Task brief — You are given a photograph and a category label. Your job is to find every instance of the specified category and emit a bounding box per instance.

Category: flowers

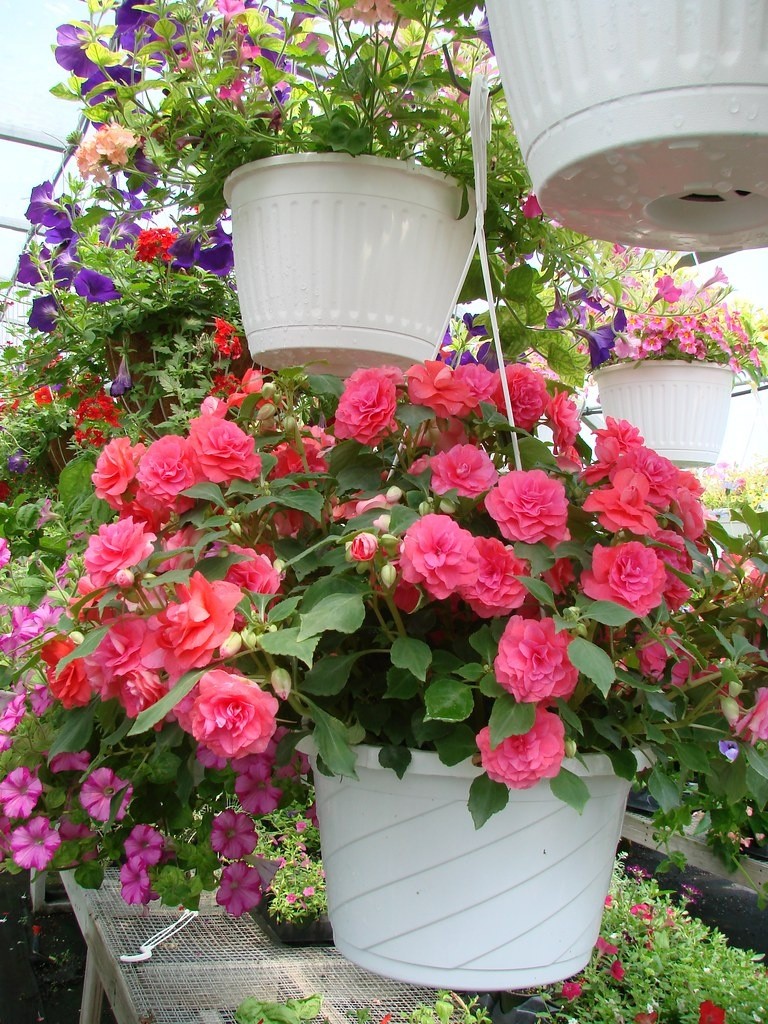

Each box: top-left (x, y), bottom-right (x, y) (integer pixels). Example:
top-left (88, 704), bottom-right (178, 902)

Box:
top-left (1, 0), bottom-right (766, 1023)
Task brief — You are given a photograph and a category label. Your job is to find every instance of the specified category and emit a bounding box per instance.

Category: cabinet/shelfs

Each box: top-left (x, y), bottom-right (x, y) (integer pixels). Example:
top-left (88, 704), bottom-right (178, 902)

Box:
top-left (625, 785), bottom-right (768, 895)
top-left (26, 859), bottom-right (483, 1023)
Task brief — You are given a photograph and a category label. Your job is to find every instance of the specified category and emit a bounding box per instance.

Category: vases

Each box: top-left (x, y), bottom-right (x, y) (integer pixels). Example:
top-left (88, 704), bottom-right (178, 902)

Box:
top-left (483, 0), bottom-right (766, 253)
top-left (222, 152), bottom-right (477, 379)
top-left (292, 732), bottom-right (659, 992)
top-left (102, 322), bottom-right (260, 442)
top-left (257, 885), bottom-right (334, 945)
top-left (46, 424), bottom-right (82, 472)
top-left (593, 358), bottom-right (735, 470)
